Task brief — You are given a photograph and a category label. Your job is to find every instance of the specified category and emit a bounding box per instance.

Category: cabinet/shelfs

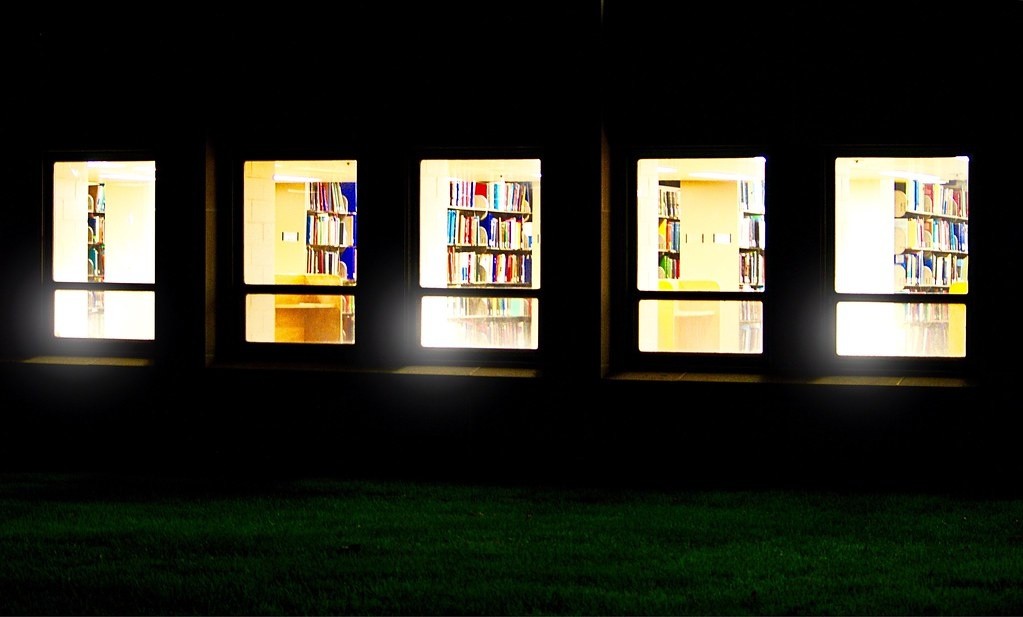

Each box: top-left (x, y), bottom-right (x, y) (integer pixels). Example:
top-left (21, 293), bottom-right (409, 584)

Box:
top-left (659, 179), bottom-right (682, 280)
top-left (892, 172), bottom-right (969, 359)
top-left (737, 177), bottom-right (764, 354)
top-left (87, 180), bottom-right (108, 319)
top-left (273, 273), bottom-right (342, 344)
top-left (448, 176), bottom-right (538, 350)
top-left (305, 180), bottom-right (357, 343)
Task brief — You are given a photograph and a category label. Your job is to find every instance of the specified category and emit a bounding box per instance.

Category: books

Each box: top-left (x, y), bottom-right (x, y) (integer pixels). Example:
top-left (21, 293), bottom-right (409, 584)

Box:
top-left (88, 184), bottom-right (104, 314)
top-left (448, 178), bottom-right (535, 343)
top-left (307, 182), bottom-right (356, 279)
top-left (891, 182), bottom-right (969, 355)
top-left (740, 182), bottom-right (765, 353)
top-left (658, 182), bottom-right (682, 286)
